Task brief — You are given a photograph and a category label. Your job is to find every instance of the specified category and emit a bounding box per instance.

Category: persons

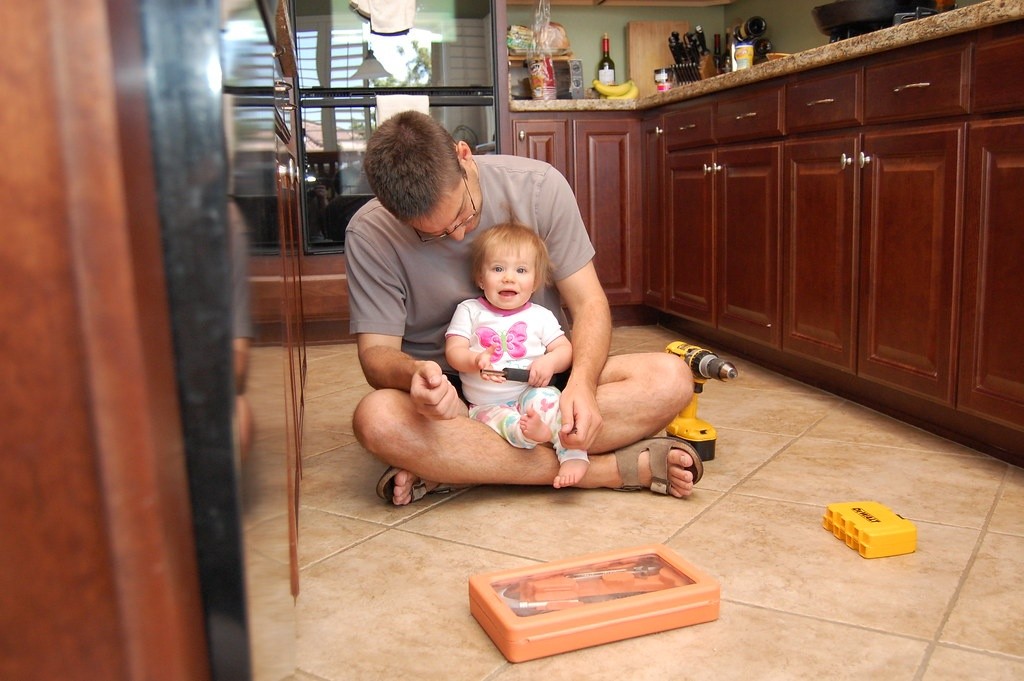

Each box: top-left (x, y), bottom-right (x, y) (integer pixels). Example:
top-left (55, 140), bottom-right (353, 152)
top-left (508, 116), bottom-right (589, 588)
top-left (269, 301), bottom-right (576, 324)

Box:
top-left (230, 197), bottom-right (255, 460)
top-left (343, 112), bottom-right (703, 504)
top-left (305, 181), bottom-right (332, 241)
top-left (445, 224), bottom-right (588, 488)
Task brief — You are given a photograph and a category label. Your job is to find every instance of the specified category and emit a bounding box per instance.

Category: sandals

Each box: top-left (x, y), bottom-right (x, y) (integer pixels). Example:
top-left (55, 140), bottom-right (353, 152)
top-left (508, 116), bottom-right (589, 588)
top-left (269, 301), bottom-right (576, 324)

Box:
top-left (377, 468), bottom-right (468, 503)
top-left (613, 437), bottom-right (704, 494)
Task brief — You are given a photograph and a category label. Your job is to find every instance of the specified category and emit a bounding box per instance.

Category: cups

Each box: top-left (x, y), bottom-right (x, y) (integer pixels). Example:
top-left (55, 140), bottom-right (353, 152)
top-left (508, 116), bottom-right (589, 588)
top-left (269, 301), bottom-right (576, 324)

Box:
top-left (655, 69), bottom-right (674, 92)
top-left (735, 42), bottom-right (753, 70)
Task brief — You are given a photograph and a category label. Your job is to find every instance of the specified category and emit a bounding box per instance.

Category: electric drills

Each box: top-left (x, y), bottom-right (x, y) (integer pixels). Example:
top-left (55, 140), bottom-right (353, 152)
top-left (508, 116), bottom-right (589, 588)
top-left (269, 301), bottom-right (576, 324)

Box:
top-left (661, 341), bottom-right (737, 462)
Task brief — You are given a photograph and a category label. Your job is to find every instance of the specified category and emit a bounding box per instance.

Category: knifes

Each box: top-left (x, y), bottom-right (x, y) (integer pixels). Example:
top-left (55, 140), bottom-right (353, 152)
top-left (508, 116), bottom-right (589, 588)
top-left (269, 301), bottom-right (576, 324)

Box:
top-left (668, 26), bottom-right (710, 85)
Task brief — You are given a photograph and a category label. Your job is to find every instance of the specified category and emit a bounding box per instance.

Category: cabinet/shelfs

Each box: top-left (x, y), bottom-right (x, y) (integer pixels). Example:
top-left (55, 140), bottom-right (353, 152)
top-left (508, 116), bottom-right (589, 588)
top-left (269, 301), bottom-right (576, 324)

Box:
top-left (507, 108), bottom-right (641, 322)
top-left (640, 16), bottom-right (1023, 471)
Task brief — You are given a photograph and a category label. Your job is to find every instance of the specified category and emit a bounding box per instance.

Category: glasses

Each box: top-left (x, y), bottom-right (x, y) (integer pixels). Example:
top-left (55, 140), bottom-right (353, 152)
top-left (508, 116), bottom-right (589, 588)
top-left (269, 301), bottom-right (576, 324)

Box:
top-left (412, 157), bottom-right (479, 242)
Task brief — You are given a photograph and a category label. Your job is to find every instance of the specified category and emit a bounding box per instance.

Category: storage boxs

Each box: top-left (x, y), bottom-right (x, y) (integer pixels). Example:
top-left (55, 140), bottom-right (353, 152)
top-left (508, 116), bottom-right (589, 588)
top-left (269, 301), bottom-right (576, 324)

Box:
top-left (467, 542), bottom-right (721, 664)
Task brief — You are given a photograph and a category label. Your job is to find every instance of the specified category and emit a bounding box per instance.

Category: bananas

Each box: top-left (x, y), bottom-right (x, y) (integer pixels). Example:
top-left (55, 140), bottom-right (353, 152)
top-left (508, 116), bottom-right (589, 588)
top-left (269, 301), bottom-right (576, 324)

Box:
top-left (593, 79), bottom-right (639, 100)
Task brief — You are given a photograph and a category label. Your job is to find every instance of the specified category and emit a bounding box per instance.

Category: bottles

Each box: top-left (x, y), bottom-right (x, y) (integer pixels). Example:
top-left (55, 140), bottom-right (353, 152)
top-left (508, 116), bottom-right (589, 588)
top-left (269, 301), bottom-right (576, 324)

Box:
top-left (599, 33), bottom-right (614, 85)
top-left (754, 39), bottom-right (772, 60)
top-left (722, 28), bottom-right (732, 73)
top-left (713, 34), bottom-right (721, 74)
top-left (734, 16), bottom-right (767, 42)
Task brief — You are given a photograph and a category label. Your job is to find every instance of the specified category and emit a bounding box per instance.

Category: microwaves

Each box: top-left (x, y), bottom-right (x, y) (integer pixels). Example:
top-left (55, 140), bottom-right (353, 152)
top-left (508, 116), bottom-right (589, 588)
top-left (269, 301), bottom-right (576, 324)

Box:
top-left (510, 57), bottom-right (583, 99)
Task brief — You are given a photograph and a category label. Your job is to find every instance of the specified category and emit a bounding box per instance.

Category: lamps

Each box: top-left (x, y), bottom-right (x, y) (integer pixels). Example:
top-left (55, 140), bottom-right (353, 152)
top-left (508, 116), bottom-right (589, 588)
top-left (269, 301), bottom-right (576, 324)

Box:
top-left (348, 33), bottom-right (390, 80)
